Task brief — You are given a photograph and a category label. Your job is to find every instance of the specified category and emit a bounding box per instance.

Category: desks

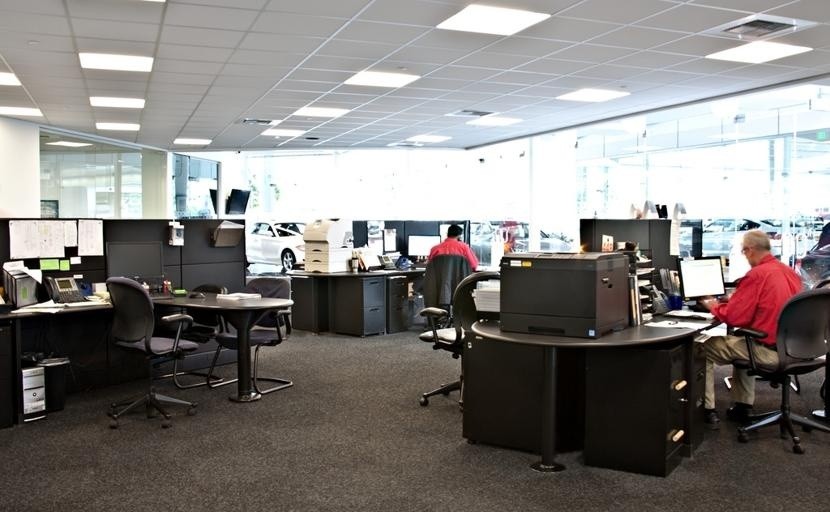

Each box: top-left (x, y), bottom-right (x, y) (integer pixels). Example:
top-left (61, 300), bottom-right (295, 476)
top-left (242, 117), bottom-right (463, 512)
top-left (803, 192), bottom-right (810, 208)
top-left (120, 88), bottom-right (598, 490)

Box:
top-left (0, 291), bottom-right (294, 429)
top-left (462, 300), bottom-right (723, 473)
top-left (287, 266), bottom-right (425, 339)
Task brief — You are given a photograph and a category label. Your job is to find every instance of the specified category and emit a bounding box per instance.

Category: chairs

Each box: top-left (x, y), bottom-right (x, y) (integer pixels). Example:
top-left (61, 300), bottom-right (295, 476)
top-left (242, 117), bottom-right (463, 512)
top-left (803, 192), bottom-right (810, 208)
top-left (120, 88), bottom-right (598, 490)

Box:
top-left (732, 285), bottom-right (830, 455)
top-left (155, 283), bottom-right (228, 389)
top-left (423, 256), bottom-right (472, 330)
top-left (106, 276), bottom-right (197, 428)
top-left (206, 276), bottom-right (294, 394)
top-left (418, 271), bottom-right (501, 408)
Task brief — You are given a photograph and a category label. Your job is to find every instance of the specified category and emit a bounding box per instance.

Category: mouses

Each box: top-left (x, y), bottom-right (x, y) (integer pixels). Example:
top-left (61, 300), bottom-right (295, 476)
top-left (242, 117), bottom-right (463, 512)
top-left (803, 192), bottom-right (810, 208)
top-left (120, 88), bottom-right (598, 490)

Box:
top-left (189, 292), bottom-right (205, 299)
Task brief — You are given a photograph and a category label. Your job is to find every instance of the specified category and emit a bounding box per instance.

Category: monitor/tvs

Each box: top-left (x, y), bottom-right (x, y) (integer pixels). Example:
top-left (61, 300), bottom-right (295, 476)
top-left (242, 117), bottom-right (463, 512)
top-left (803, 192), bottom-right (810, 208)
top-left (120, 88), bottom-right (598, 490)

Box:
top-left (676, 255), bottom-right (725, 311)
top-left (106, 240), bottom-right (164, 289)
top-left (226, 188), bottom-right (251, 214)
top-left (408, 234), bottom-right (441, 261)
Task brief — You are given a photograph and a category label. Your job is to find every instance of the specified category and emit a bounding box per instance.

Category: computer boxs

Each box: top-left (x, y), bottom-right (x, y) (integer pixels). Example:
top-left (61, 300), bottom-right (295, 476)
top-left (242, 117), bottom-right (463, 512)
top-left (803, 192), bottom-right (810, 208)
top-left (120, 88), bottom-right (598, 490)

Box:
top-left (21, 366), bottom-right (47, 423)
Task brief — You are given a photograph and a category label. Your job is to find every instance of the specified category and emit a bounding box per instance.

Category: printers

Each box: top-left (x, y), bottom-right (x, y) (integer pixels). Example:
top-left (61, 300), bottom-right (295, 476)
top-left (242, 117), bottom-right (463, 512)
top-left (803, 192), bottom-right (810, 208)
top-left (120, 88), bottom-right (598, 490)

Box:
top-left (498, 252), bottom-right (631, 340)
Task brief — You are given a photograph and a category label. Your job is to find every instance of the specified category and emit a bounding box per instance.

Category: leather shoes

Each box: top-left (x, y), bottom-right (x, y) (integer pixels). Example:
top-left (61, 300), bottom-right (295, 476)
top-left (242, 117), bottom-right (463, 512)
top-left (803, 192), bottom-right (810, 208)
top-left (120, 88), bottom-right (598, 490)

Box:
top-left (727, 405), bottom-right (751, 418)
top-left (705, 413), bottom-right (721, 431)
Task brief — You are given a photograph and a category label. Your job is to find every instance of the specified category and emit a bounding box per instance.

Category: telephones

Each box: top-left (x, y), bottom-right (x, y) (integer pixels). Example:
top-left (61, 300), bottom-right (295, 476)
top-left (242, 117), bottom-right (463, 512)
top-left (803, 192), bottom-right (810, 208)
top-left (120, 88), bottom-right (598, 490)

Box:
top-left (640, 284), bottom-right (669, 315)
top-left (378, 255), bottom-right (397, 270)
top-left (43, 275), bottom-right (85, 303)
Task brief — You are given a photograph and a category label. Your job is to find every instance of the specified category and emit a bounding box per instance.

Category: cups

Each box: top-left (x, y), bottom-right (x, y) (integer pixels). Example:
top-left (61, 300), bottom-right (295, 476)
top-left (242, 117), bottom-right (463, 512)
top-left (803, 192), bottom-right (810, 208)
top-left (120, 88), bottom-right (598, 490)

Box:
top-left (667, 294), bottom-right (682, 310)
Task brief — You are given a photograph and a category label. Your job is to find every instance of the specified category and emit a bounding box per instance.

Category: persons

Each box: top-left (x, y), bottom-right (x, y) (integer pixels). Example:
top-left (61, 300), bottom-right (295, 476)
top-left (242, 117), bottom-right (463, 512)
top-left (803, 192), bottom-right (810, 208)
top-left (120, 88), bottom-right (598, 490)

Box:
top-left (703, 229), bottom-right (804, 431)
top-left (429, 225), bottom-right (480, 269)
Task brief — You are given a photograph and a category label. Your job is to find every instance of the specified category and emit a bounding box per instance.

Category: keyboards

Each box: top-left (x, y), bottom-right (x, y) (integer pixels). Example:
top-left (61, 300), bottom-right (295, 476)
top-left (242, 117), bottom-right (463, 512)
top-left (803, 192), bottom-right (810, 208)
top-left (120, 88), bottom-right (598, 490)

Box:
top-left (149, 292), bottom-right (173, 300)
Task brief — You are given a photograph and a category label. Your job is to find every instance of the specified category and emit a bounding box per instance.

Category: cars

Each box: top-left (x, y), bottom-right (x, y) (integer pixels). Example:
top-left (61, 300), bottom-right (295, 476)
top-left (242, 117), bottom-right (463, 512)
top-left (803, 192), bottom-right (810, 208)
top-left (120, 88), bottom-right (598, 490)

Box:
top-left (469, 222), bottom-right (582, 265)
top-left (677, 215), bottom-right (830, 289)
top-left (246, 220), bottom-right (315, 271)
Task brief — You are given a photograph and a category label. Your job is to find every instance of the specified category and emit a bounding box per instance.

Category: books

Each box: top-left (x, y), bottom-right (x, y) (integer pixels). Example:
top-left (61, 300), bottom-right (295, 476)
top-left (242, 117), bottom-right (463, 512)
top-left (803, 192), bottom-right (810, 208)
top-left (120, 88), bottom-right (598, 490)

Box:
top-left (629, 267), bottom-right (655, 326)
top-left (471, 289), bottom-right (501, 312)
top-left (217, 291), bottom-right (260, 301)
